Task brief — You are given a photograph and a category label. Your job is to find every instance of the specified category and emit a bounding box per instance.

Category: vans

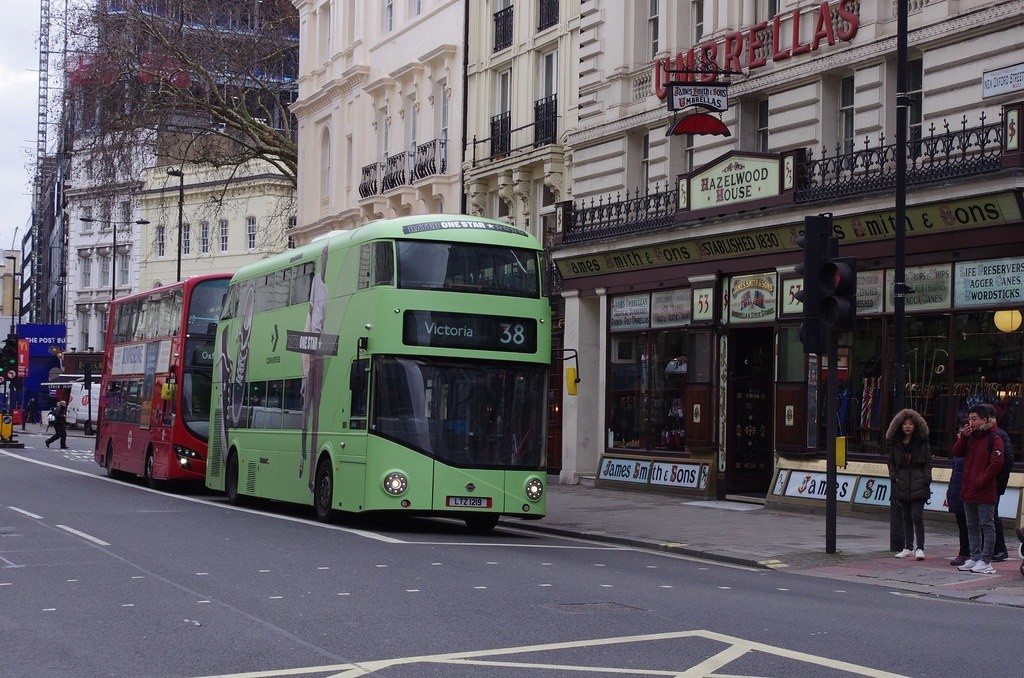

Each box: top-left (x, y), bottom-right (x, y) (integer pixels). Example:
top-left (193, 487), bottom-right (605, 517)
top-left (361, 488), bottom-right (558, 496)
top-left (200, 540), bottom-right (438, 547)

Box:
top-left (65, 381), bottom-right (101, 431)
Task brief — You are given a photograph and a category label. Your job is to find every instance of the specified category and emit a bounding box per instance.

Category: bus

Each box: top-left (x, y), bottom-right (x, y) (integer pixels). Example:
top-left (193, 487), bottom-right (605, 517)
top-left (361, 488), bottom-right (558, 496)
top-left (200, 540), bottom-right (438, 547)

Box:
top-left (94, 272), bottom-right (234, 491)
top-left (206, 214), bottom-right (581, 534)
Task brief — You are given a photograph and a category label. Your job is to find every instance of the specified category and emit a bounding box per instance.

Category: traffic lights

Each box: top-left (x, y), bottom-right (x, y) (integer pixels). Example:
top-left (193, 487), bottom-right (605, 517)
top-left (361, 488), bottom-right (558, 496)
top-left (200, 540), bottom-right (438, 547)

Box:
top-left (6, 339), bottom-right (18, 369)
top-left (819, 256), bottom-right (857, 332)
top-left (0, 359), bottom-right (6, 375)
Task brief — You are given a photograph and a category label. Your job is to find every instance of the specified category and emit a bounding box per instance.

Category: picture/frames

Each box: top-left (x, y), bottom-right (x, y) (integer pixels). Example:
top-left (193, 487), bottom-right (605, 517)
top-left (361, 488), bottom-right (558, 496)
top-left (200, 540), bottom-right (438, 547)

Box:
top-left (615, 337), bottom-right (635, 363)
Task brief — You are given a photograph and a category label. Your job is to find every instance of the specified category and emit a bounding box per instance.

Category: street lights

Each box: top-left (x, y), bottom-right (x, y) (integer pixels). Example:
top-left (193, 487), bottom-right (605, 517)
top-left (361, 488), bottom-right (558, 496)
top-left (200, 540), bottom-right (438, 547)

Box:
top-left (6, 254), bottom-right (17, 333)
top-left (80, 217), bottom-right (151, 302)
top-left (166, 170), bottom-right (184, 283)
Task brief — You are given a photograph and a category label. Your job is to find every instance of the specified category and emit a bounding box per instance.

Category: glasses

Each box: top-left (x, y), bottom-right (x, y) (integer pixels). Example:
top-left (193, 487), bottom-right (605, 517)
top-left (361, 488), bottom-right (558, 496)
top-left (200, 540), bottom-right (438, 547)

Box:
top-left (968, 418), bottom-right (982, 421)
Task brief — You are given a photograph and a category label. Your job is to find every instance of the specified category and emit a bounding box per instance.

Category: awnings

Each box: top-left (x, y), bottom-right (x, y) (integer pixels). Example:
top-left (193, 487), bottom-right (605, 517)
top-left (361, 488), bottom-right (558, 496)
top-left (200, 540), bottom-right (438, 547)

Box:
top-left (42, 373), bottom-right (102, 386)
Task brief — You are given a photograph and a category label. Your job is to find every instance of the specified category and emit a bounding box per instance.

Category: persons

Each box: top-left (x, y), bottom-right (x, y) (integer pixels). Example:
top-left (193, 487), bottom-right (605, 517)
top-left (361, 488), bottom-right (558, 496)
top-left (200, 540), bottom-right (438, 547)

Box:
top-left (192, 394), bottom-right (203, 410)
top-left (2, 398), bottom-right (36, 424)
top-left (946, 404), bottom-right (1013, 574)
top-left (885, 408), bottom-right (933, 561)
top-left (55, 385), bottom-right (64, 401)
top-left (45, 401), bottom-right (69, 449)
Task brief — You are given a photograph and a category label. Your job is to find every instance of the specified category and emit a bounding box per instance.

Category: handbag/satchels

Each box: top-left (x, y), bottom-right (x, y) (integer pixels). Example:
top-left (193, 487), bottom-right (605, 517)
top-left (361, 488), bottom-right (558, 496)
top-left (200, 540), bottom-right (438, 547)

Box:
top-left (47, 413), bottom-right (57, 426)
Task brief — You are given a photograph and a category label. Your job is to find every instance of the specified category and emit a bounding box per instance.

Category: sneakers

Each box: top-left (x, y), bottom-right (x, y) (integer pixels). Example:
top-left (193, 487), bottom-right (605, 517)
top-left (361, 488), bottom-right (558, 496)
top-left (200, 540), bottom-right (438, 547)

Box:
top-left (950, 556), bottom-right (969, 565)
top-left (970, 560), bottom-right (992, 572)
top-left (916, 549), bottom-right (925, 559)
top-left (990, 551), bottom-right (1008, 561)
top-left (61, 446), bottom-right (69, 449)
top-left (895, 549), bottom-right (915, 558)
top-left (958, 559), bottom-right (976, 570)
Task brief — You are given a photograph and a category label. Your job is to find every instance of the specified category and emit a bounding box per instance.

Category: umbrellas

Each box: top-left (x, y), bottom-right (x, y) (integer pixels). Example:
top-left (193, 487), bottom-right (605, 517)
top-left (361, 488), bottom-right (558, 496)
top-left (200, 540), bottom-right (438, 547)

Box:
top-left (838, 375), bottom-right (1023, 450)
top-left (614, 395), bottom-right (684, 447)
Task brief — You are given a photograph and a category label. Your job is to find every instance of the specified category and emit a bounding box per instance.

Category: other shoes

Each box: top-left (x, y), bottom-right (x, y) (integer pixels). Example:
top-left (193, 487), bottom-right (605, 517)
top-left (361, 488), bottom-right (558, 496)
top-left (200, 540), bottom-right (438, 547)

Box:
top-left (45, 440), bottom-right (49, 447)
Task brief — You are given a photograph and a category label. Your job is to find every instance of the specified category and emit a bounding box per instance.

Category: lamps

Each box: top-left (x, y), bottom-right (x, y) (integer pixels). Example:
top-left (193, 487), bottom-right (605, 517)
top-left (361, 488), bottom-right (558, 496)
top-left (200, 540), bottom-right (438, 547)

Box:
top-left (994, 309), bottom-right (1022, 333)
